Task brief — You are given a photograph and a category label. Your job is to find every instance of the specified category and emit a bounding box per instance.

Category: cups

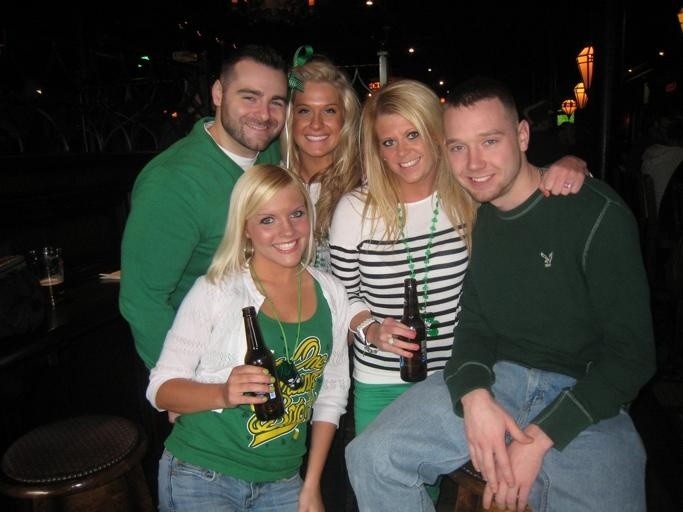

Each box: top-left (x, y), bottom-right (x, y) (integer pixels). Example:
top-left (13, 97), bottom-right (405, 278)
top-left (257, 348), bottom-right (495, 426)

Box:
top-left (27, 247), bottom-right (65, 306)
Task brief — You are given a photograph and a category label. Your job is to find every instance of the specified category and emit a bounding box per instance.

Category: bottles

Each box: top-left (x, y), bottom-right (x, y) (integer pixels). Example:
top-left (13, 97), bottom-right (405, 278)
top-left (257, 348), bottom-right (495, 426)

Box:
top-left (242, 306), bottom-right (284, 422)
top-left (399, 278), bottom-right (427, 383)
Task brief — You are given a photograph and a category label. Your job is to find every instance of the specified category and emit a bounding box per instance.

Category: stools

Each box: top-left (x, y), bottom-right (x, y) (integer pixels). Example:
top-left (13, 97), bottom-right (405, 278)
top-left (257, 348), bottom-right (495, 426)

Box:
top-left (0, 412), bottom-right (158, 512)
top-left (447, 463), bottom-right (536, 512)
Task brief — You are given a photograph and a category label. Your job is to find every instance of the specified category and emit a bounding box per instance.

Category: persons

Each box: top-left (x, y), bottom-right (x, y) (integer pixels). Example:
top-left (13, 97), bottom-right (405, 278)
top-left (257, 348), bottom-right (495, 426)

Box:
top-left (115, 47), bottom-right (683, 512)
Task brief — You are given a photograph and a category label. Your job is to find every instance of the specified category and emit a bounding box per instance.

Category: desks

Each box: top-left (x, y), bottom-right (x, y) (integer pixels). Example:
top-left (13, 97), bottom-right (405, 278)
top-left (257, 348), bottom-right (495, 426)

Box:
top-left (0, 257), bottom-right (120, 368)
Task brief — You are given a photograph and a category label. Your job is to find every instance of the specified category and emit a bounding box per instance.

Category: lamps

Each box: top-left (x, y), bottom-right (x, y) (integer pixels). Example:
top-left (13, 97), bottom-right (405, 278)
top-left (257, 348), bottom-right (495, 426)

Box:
top-left (575, 40), bottom-right (601, 92)
top-left (561, 99), bottom-right (577, 121)
top-left (573, 82), bottom-right (589, 111)
